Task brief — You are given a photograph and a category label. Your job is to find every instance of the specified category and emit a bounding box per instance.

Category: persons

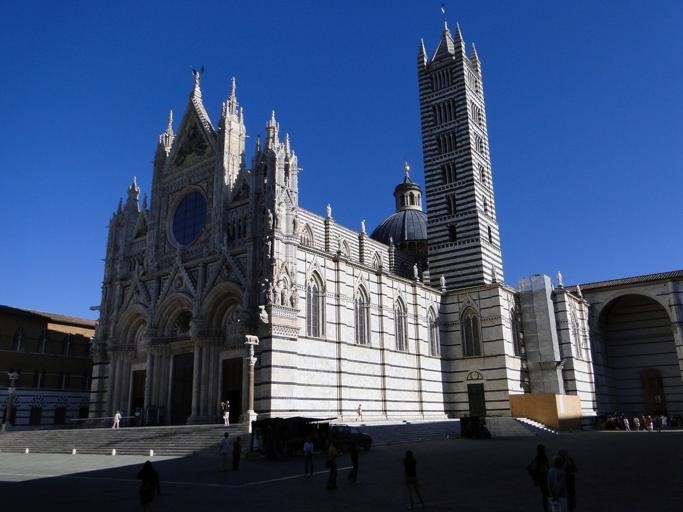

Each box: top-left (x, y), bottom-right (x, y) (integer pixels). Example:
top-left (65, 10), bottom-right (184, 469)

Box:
top-left (403, 450), bottom-right (424, 509)
top-left (113, 410), bottom-right (122, 430)
top-left (218, 432), bottom-right (229, 472)
top-left (300, 434), bottom-right (361, 489)
top-left (233, 437), bottom-right (242, 470)
top-left (530, 443), bottom-right (577, 511)
top-left (136, 461), bottom-right (160, 512)
top-left (221, 401), bottom-right (230, 426)
top-left (605, 411), bottom-right (669, 432)
top-left (355, 404), bottom-right (363, 421)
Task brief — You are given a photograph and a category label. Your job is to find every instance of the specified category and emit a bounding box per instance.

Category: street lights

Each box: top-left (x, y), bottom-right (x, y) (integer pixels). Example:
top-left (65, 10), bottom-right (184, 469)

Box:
top-left (243, 333), bottom-right (262, 435)
top-left (1, 370), bottom-right (21, 434)
